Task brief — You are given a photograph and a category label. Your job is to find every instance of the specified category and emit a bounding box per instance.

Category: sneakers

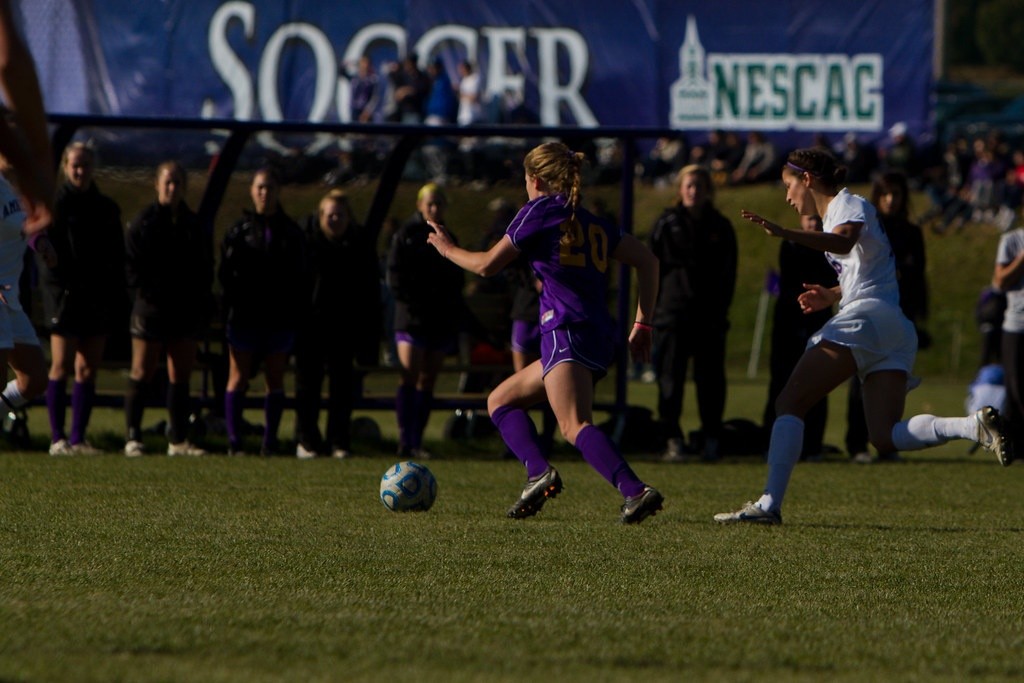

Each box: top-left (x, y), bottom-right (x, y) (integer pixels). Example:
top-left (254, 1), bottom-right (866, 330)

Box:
top-left (506, 465), bottom-right (563, 518)
top-left (621, 485), bottom-right (662, 523)
top-left (978, 406), bottom-right (1013, 466)
top-left (713, 501), bottom-right (782, 524)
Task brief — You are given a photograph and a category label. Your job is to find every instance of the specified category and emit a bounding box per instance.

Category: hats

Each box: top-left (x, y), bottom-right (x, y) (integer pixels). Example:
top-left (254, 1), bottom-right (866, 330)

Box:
top-left (889, 122), bottom-right (908, 136)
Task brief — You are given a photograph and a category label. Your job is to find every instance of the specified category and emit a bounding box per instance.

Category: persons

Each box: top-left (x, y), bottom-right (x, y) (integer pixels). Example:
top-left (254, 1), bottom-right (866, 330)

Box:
top-left (424, 139), bottom-right (666, 524)
top-left (714, 145), bottom-right (1014, 526)
top-left (1, 51), bottom-right (1024, 463)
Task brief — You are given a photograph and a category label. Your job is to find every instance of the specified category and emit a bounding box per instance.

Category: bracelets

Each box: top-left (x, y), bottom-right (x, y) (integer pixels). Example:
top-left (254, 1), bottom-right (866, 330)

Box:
top-left (634, 319), bottom-right (653, 327)
top-left (633, 321), bottom-right (653, 331)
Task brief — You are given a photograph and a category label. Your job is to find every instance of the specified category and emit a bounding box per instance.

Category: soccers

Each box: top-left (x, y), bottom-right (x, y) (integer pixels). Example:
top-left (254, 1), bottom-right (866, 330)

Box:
top-left (379, 461), bottom-right (437, 514)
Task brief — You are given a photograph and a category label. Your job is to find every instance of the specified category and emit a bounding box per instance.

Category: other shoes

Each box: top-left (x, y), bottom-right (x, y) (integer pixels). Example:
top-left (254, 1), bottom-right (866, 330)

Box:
top-left (848, 447), bottom-right (872, 463)
top-left (49, 439), bottom-right (104, 456)
top-left (297, 443), bottom-right (349, 459)
top-left (397, 447), bottom-right (432, 459)
top-left (168, 438), bottom-right (205, 457)
top-left (227, 440), bottom-right (280, 460)
top-left (125, 441), bottom-right (145, 458)
top-left (664, 438), bottom-right (723, 461)
